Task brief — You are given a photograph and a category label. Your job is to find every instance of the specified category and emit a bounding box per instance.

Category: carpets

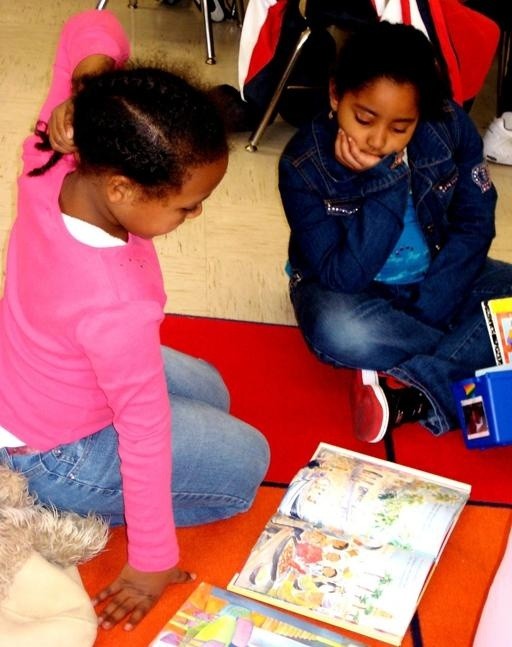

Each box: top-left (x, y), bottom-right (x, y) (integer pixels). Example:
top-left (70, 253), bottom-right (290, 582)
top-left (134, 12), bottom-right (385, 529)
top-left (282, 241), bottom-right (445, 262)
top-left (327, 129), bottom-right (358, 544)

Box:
top-left (80, 307), bottom-right (511, 646)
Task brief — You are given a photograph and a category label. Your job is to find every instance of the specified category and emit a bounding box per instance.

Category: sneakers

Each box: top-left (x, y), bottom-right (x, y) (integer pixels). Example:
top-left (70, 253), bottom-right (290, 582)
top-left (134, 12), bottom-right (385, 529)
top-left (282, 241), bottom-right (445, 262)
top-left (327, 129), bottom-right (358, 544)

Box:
top-left (352, 366), bottom-right (423, 443)
top-left (481, 116), bottom-right (512, 165)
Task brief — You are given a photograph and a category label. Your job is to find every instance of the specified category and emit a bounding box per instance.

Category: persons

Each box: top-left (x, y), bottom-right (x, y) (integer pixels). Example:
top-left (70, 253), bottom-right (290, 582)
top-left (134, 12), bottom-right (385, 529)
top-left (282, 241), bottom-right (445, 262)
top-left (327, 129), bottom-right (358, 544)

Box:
top-left (280, 24), bottom-right (512, 446)
top-left (373, 1), bottom-right (512, 169)
top-left (0, 8), bottom-right (270, 633)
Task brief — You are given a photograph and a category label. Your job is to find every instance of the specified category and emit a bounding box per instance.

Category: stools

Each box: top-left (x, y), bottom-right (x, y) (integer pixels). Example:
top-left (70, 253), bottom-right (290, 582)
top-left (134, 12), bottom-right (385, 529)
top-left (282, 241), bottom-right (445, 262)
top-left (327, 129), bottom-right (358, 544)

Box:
top-left (95, 0), bottom-right (217, 66)
top-left (243, 24), bottom-right (328, 154)
